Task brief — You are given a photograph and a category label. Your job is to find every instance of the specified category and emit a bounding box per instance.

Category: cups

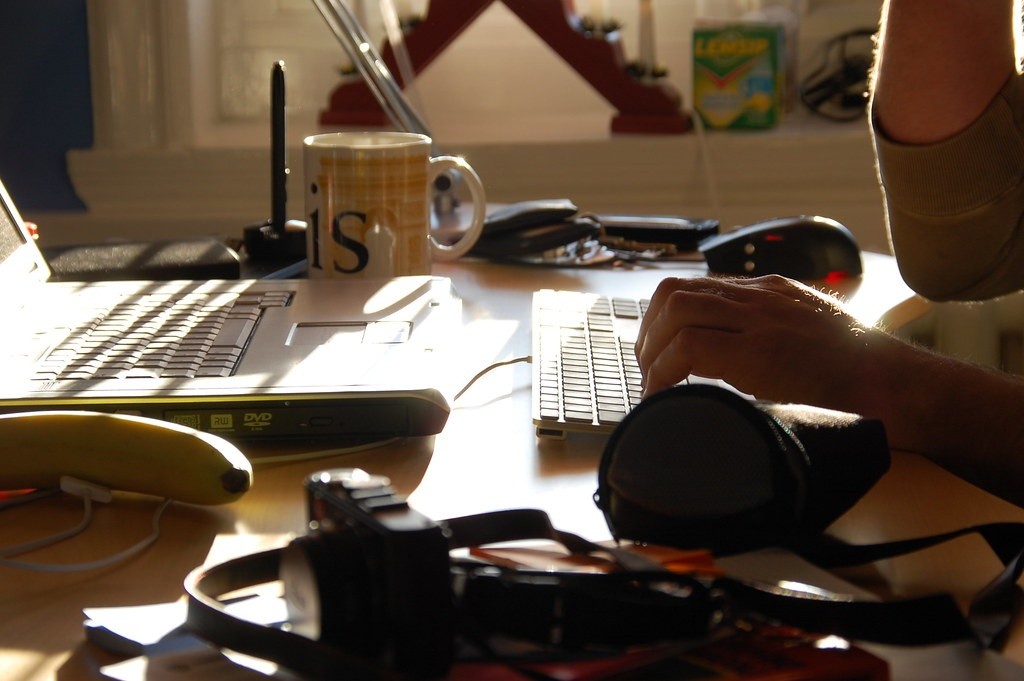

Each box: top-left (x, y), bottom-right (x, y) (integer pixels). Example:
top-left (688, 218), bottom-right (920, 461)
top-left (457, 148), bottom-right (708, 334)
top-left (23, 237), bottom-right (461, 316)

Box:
top-left (303, 132), bottom-right (487, 282)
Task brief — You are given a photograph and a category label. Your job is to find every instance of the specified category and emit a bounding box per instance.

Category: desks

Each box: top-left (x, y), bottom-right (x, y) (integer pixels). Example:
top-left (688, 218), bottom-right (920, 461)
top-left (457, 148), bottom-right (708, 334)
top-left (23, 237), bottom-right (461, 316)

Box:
top-left (0, 249), bottom-right (1024, 681)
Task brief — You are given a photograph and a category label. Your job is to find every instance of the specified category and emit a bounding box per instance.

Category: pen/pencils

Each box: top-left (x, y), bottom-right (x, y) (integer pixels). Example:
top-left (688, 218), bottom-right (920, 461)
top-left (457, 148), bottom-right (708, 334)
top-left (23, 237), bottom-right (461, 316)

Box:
top-left (270, 60), bottom-right (289, 230)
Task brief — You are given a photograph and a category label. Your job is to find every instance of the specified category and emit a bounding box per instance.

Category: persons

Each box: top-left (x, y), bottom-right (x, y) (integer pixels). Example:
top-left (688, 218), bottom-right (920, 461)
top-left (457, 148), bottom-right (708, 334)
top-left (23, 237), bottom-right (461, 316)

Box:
top-left (633, 0), bottom-right (1024, 509)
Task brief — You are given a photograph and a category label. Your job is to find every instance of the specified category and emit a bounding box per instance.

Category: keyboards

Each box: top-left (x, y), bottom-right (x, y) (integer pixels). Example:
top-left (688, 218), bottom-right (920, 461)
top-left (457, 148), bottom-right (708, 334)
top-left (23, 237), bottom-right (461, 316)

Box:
top-left (531, 288), bottom-right (760, 441)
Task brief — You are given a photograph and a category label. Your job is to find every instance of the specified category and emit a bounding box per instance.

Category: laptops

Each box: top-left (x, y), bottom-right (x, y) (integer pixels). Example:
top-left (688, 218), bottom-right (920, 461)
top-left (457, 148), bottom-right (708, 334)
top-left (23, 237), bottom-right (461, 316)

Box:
top-left (0, 182), bottom-right (463, 448)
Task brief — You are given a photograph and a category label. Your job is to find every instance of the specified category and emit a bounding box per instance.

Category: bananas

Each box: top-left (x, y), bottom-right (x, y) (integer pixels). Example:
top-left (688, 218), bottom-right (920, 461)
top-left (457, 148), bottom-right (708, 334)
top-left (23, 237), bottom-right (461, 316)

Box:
top-left (0, 410), bottom-right (252, 505)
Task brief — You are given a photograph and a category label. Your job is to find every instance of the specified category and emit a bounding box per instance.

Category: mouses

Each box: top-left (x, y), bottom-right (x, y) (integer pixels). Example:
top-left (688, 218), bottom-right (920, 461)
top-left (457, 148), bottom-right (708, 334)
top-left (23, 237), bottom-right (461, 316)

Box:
top-left (699, 215), bottom-right (862, 282)
